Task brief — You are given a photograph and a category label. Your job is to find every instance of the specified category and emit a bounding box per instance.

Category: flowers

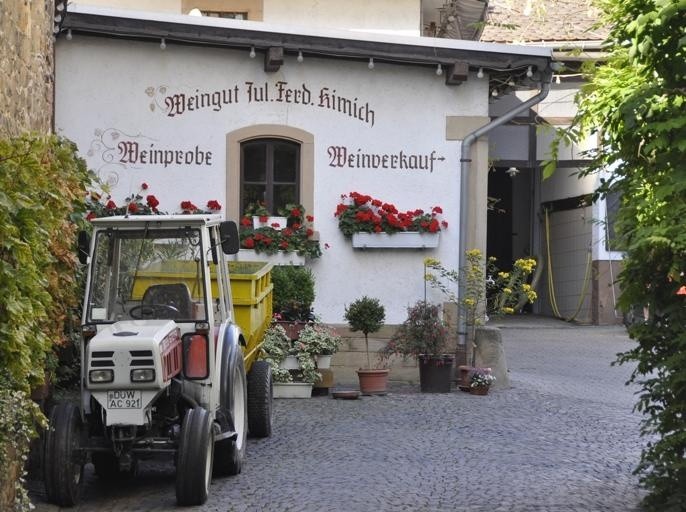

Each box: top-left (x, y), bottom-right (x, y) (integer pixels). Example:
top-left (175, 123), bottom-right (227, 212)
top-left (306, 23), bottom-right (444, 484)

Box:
top-left (370, 248), bottom-right (539, 389)
top-left (236, 202), bottom-right (324, 256)
top-left (333, 191), bottom-right (448, 235)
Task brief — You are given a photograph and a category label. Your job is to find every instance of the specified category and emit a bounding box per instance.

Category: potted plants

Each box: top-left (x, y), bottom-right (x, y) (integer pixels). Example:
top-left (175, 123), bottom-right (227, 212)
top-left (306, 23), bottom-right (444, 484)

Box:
top-left (255, 263), bottom-right (342, 399)
top-left (342, 296), bottom-right (390, 395)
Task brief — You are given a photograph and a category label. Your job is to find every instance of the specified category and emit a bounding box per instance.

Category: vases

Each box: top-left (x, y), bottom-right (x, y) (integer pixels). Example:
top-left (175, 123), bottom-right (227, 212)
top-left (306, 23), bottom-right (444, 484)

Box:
top-left (455, 364), bottom-right (477, 393)
top-left (469, 384), bottom-right (489, 395)
top-left (418, 355), bottom-right (454, 394)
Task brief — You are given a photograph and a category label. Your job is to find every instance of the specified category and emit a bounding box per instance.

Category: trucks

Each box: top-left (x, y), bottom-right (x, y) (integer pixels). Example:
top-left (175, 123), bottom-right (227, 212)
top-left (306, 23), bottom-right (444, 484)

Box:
top-left (42, 214), bottom-right (273, 506)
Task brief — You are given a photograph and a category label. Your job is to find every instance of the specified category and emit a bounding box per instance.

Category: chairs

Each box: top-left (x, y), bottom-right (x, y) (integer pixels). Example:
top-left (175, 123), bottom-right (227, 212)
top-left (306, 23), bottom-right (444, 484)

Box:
top-left (139, 282), bottom-right (192, 321)
